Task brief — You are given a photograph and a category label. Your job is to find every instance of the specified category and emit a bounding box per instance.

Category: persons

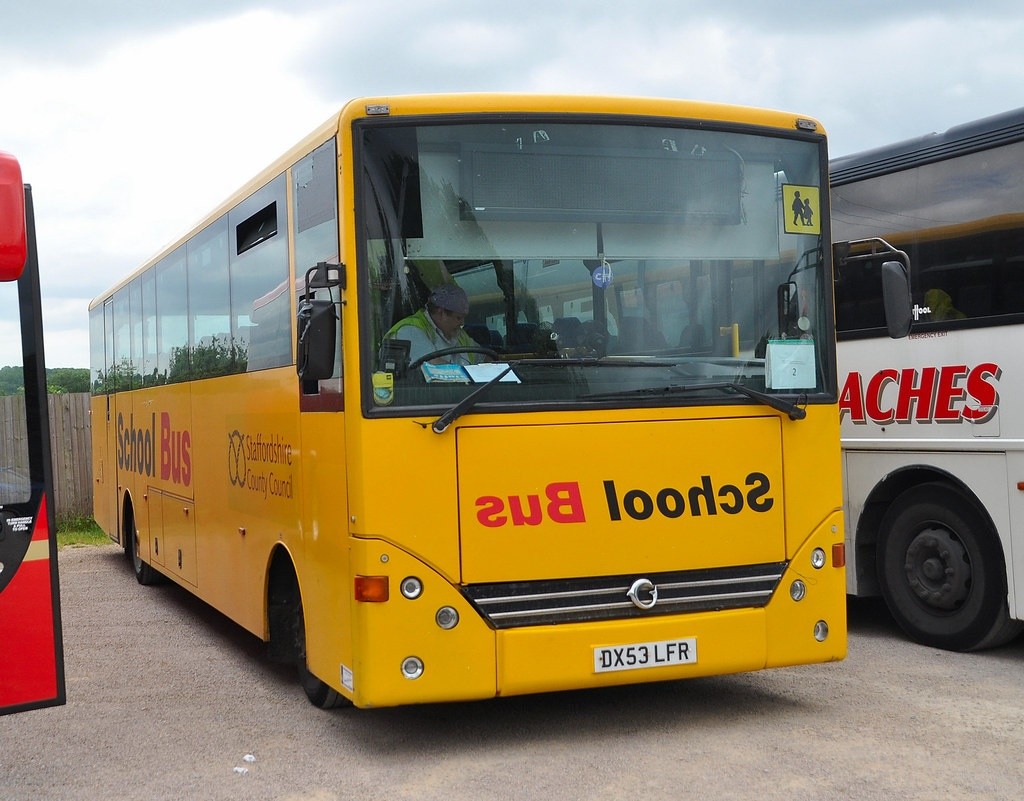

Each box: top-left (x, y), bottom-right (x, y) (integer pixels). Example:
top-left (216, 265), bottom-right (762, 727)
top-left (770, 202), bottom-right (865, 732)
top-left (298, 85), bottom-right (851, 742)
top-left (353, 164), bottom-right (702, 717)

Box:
top-left (378, 281), bottom-right (494, 370)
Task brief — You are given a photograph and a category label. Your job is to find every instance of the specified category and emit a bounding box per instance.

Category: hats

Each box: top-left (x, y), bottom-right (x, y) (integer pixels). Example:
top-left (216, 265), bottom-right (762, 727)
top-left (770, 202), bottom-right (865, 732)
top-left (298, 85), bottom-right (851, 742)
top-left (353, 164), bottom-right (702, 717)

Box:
top-left (429, 283), bottom-right (469, 315)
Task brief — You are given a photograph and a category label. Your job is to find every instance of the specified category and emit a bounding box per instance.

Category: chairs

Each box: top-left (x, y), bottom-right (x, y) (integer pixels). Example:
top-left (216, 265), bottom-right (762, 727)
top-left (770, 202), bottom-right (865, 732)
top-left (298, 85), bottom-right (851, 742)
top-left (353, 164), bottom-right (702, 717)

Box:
top-left (464, 317), bottom-right (625, 358)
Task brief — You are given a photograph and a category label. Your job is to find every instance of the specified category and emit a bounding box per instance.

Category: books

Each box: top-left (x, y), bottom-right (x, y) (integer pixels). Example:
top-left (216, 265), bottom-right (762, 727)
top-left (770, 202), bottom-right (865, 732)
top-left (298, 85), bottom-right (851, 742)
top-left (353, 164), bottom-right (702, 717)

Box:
top-left (420, 361), bottom-right (471, 383)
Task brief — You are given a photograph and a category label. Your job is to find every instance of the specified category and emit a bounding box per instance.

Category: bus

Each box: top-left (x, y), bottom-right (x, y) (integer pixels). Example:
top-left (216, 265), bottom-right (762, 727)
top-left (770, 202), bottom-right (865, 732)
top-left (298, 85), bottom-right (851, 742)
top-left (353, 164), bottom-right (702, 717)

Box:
top-left (0, 152), bottom-right (67, 715)
top-left (246, 252), bottom-right (338, 414)
top-left (453, 104), bottom-right (1024, 653)
top-left (88, 92), bottom-right (920, 711)
